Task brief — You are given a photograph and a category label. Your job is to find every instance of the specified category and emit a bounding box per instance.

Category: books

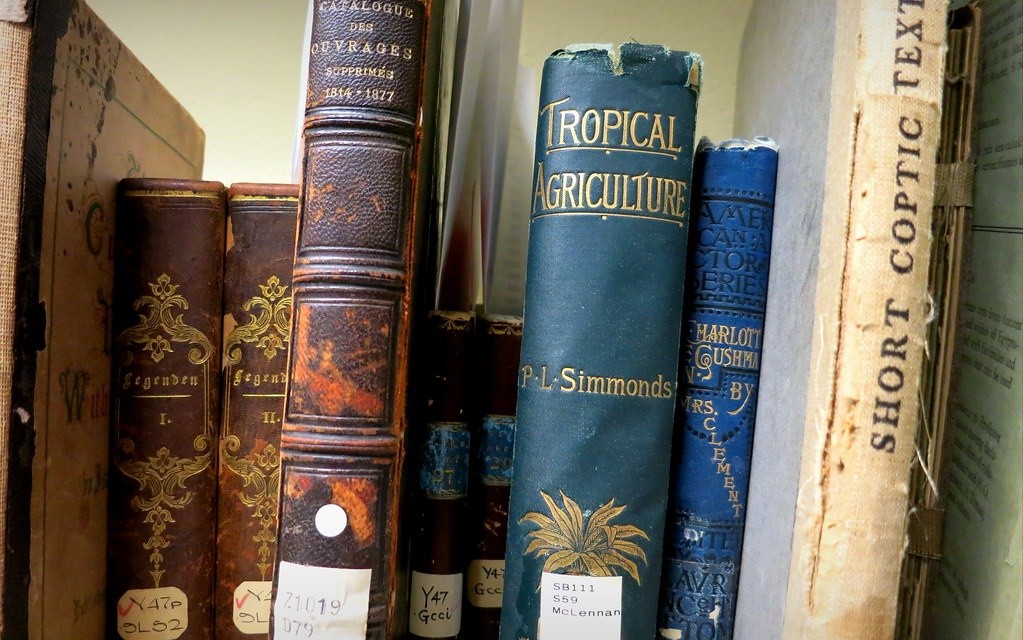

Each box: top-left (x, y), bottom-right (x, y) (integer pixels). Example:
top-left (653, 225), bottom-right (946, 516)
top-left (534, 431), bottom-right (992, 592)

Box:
top-left (1, 0), bottom-right (1023, 640)
